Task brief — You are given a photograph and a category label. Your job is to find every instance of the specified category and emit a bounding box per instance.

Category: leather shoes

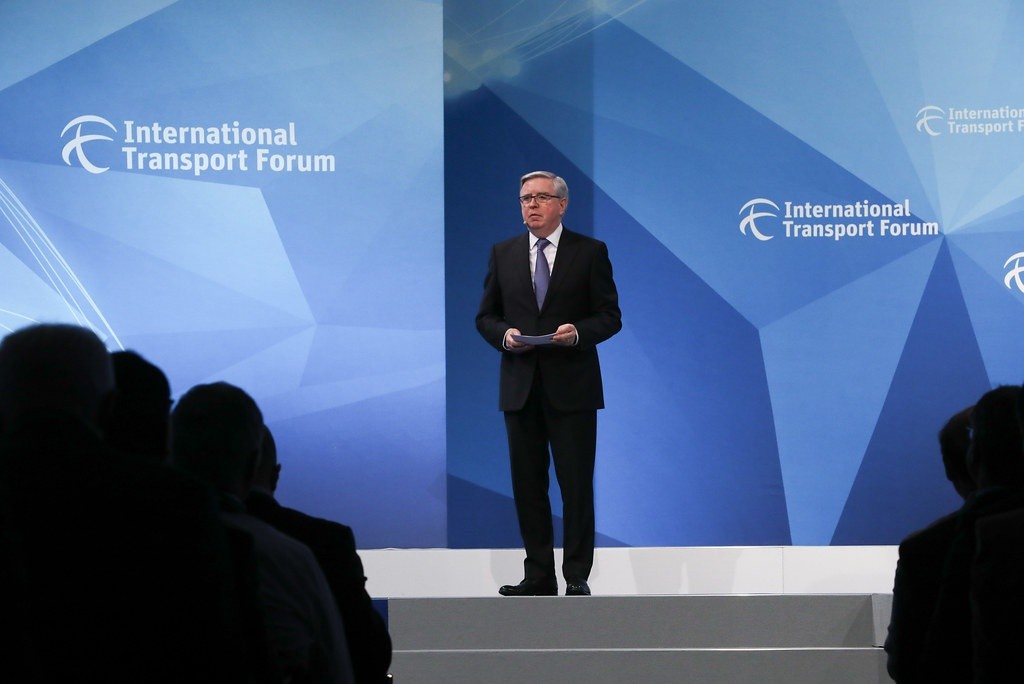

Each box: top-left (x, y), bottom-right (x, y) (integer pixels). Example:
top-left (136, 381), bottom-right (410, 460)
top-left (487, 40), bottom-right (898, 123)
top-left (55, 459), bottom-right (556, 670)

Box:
top-left (565, 578), bottom-right (591, 596)
top-left (498, 577), bottom-right (558, 596)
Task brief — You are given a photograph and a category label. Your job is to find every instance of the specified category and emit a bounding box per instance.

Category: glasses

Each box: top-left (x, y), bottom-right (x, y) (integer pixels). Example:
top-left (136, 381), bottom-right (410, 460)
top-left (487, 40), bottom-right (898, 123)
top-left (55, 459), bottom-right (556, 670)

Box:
top-left (518, 193), bottom-right (562, 204)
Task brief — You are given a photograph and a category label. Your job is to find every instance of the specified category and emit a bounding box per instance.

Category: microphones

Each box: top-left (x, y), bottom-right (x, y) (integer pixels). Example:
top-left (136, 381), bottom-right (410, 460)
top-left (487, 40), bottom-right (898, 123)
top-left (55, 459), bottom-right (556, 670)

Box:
top-left (523, 221), bottom-right (527, 224)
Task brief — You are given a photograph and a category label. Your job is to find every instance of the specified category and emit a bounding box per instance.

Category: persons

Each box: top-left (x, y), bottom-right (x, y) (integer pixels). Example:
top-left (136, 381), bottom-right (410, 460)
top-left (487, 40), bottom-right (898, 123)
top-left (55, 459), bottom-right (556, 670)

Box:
top-left (0, 326), bottom-right (392, 684)
top-left (885, 384), bottom-right (1024, 684)
top-left (473, 169), bottom-right (623, 595)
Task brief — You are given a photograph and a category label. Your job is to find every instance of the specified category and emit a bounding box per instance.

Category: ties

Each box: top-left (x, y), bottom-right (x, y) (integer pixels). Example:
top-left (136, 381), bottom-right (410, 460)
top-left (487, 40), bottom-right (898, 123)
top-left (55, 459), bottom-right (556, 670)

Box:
top-left (534, 239), bottom-right (551, 311)
